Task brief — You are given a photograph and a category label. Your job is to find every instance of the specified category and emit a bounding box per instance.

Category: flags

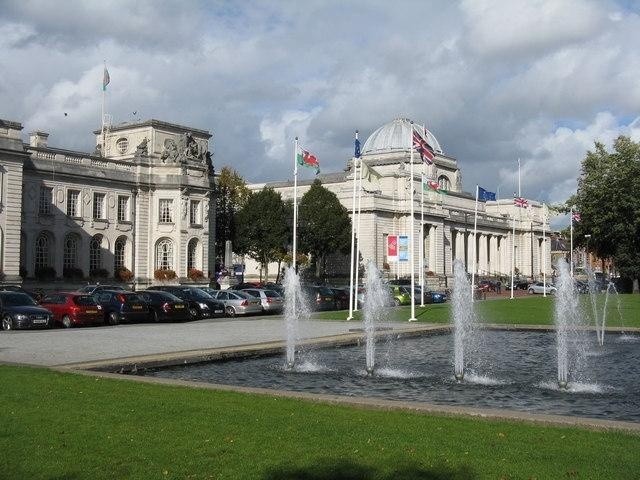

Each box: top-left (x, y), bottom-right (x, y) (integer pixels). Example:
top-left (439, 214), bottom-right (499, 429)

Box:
top-left (355, 134), bottom-right (361, 159)
top-left (412, 126), bottom-right (435, 166)
top-left (362, 161), bottom-right (383, 185)
top-left (423, 175), bottom-right (447, 195)
top-left (297, 143), bottom-right (320, 175)
top-left (478, 186), bottom-right (496, 201)
top-left (514, 196), bottom-right (528, 209)
top-left (103, 63), bottom-right (110, 91)
top-left (572, 211), bottom-right (580, 219)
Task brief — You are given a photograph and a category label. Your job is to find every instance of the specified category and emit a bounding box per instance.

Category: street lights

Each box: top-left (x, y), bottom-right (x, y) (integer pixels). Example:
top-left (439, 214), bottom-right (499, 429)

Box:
top-left (584, 234), bottom-right (591, 264)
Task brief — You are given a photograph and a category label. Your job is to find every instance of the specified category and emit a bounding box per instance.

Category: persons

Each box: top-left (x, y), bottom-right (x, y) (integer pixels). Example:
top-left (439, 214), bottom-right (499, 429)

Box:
top-left (496, 280), bottom-right (501, 294)
